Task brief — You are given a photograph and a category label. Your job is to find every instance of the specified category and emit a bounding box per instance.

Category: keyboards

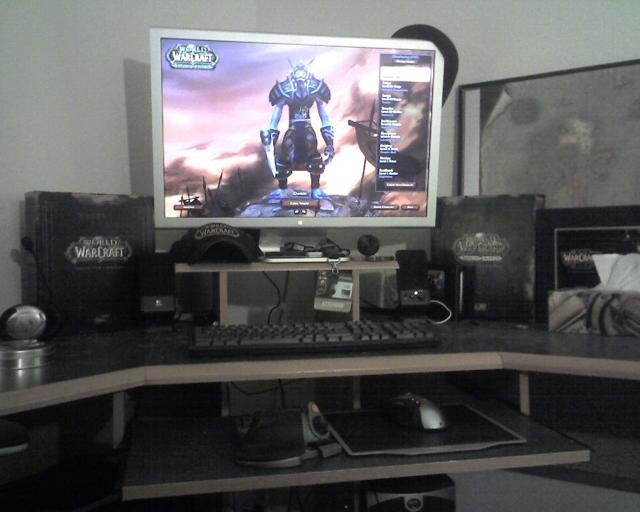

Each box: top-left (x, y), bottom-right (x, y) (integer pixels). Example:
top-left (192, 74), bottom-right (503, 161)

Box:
top-left (192, 317), bottom-right (435, 357)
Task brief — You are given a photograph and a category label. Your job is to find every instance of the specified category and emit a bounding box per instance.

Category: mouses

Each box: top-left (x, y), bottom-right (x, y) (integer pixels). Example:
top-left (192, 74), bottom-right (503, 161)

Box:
top-left (382, 391), bottom-right (449, 430)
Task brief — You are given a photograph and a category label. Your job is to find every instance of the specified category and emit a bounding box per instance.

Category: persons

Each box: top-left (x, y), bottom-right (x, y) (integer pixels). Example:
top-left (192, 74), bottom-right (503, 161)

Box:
top-left (262, 62), bottom-right (336, 201)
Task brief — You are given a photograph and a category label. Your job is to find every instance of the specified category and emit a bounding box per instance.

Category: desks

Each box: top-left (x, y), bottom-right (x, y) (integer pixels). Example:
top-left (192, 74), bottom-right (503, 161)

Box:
top-left (0, 321), bottom-right (640, 512)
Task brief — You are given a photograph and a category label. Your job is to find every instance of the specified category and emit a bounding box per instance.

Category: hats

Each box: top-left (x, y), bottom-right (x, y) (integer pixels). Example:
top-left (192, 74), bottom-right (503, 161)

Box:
top-left (182, 223), bottom-right (255, 262)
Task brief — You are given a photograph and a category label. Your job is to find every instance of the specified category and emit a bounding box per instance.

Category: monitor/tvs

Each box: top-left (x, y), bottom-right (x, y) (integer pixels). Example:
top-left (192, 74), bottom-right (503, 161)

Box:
top-left (148, 26), bottom-right (444, 265)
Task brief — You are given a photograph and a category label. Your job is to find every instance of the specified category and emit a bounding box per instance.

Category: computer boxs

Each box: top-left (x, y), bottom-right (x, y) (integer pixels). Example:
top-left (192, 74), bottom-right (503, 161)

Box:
top-left (365, 473), bottom-right (456, 511)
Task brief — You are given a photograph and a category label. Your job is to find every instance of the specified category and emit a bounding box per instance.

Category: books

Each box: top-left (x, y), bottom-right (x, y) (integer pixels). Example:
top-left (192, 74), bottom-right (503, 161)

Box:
top-left (23, 190), bottom-right (156, 334)
top-left (430, 192), bottom-right (544, 321)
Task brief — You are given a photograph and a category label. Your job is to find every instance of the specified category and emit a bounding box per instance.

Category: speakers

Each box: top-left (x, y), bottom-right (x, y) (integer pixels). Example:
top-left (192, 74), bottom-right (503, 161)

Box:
top-left (138, 251), bottom-right (177, 335)
top-left (395, 249), bottom-right (431, 320)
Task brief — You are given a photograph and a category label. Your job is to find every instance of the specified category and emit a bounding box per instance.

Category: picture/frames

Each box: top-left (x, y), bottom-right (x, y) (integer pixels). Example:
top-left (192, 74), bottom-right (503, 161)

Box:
top-left (534, 204), bottom-right (640, 336)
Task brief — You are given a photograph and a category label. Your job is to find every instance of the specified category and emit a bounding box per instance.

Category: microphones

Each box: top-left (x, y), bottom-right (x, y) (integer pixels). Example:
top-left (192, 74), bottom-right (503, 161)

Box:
top-left (20, 234), bottom-right (40, 307)
